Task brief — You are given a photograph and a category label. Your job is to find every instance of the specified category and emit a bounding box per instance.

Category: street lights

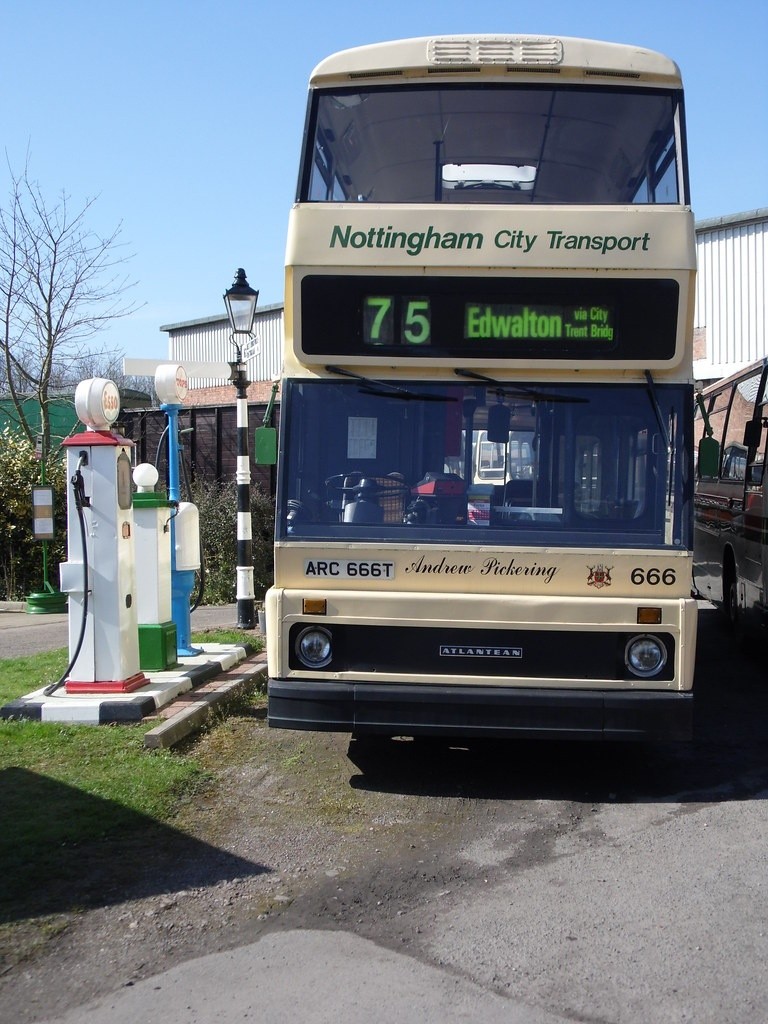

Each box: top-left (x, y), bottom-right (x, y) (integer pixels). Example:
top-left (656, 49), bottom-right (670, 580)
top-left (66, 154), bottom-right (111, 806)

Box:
top-left (218, 269), bottom-right (261, 631)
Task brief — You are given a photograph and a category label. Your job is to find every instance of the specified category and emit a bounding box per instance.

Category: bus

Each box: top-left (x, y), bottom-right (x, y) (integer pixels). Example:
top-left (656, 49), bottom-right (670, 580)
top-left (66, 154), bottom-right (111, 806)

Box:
top-left (665, 354), bottom-right (768, 657)
top-left (255, 31), bottom-right (720, 746)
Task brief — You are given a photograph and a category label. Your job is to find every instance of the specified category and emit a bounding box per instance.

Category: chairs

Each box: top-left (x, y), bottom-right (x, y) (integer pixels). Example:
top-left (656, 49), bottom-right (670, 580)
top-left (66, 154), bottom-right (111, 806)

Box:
top-left (341, 470), bottom-right (407, 523)
top-left (489, 476), bottom-right (557, 529)
